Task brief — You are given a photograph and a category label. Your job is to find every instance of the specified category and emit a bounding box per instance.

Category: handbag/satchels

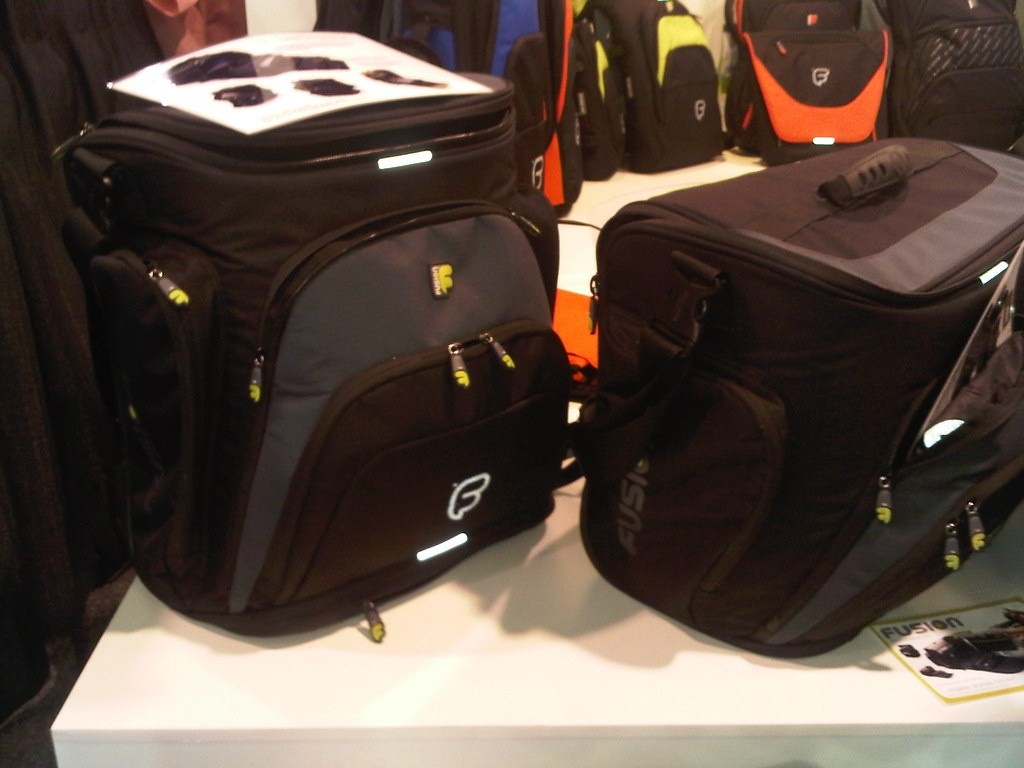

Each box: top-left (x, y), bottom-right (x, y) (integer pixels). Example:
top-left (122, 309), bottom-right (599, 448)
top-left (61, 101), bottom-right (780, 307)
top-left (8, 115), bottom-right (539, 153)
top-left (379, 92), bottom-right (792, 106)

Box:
top-left (581, 137), bottom-right (1024, 660)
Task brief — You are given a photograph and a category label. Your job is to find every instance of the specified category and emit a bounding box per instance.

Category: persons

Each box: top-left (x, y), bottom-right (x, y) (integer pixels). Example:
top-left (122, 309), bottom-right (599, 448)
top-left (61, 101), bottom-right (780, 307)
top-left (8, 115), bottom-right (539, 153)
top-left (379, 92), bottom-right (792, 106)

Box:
top-left (928, 608), bottom-right (1024, 674)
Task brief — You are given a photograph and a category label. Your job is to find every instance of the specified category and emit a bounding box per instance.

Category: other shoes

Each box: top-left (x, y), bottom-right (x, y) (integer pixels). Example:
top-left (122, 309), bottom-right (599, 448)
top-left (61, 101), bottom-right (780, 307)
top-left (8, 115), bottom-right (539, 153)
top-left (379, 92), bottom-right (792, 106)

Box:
top-left (0, 662), bottom-right (58, 730)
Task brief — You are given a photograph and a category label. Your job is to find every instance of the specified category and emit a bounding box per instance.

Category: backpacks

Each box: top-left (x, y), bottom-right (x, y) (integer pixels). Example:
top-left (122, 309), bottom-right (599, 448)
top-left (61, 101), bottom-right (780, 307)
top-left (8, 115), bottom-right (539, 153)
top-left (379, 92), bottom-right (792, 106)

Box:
top-left (57, 71), bottom-right (567, 640)
top-left (315, 0), bottom-right (1024, 216)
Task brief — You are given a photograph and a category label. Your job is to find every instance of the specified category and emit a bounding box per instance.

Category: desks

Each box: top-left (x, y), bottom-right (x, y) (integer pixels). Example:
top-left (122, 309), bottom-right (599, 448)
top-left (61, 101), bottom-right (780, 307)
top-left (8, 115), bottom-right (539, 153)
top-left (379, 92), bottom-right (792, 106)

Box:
top-left (46, 151), bottom-right (1024, 768)
